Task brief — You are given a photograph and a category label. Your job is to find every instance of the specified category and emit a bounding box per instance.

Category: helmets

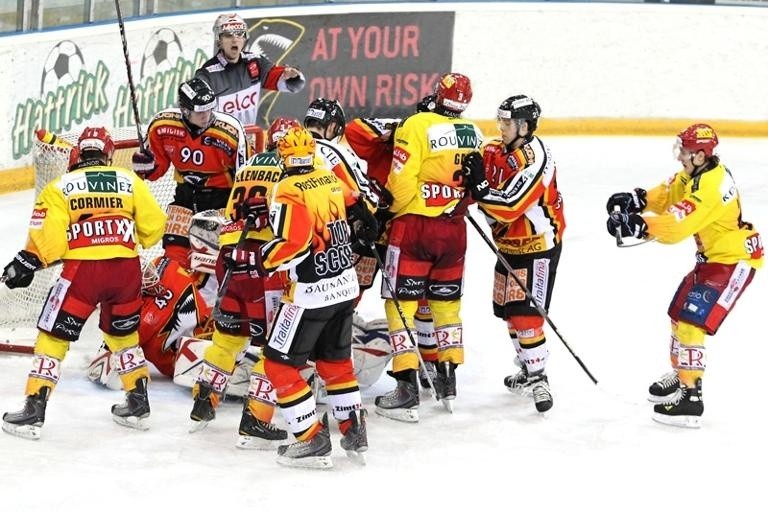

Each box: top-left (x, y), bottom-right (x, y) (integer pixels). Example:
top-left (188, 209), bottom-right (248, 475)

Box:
top-left (178, 78), bottom-right (217, 114)
top-left (433, 72), bottom-right (473, 112)
top-left (78, 127), bottom-right (115, 159)
top-left (496, 94), bottom-right (542, 125)
top-left (269, 97), bottom-right (346, 168)
top-left (672, 124), bottom-right (718, 156)
top-left (214, 13), bottom-right (249, 40)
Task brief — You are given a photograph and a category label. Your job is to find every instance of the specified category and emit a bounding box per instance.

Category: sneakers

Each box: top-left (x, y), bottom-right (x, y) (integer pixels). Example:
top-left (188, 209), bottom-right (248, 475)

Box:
top-left (649, 373), bottom-right (679, 396)
top-left (4, 386), bottom-right (51, 427)
top-left (191, 361), bottom-right (455, 457)
top-left (654, 387), bottom-right (703, 415)
top-left (504, 372), bottom-right (531, 388)
top-left (111, 377), bottom-right (151, 417)
top-left (526, 376), bottom-right (552, 412)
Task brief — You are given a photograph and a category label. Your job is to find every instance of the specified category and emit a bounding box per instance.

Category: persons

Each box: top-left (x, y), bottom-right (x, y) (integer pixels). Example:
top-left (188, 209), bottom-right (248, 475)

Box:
top-left (194, 11), bottom-right (309, 158)
top-left (84, 71), bottom-right (490, 468)
top-left (1, 125), bottom-right (170, 426)
top-left (460, 93), bottom-right (568, 414)
top-left (604, 123), bottom-right (767, 418)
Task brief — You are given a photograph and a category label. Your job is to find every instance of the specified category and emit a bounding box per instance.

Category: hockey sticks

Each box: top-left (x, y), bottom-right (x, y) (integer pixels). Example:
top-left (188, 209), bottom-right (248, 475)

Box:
top-left (369, 241), bottom-right (442, 404)
top-left (614, 204), bottom-right (659, 249)
top-left (209, 215), bottom-right (253, 325)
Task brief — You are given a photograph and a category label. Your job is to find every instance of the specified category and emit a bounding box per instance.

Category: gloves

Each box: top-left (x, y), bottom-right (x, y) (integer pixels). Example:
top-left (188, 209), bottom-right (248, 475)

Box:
top-left (222, 244), bottom-right (255, 269)
top-left (607, 188), bottom-right (649, 239)
top-left (461, 151), bottom-right (489, 199)
top-left (2, 250), bottom-right (41, 289)
top-left (356, 219), bottom-right (386, 244)
top-left (243, 197), bottom-right (268, 231)
top-left (133, 149), bottom-right (155, 173)
